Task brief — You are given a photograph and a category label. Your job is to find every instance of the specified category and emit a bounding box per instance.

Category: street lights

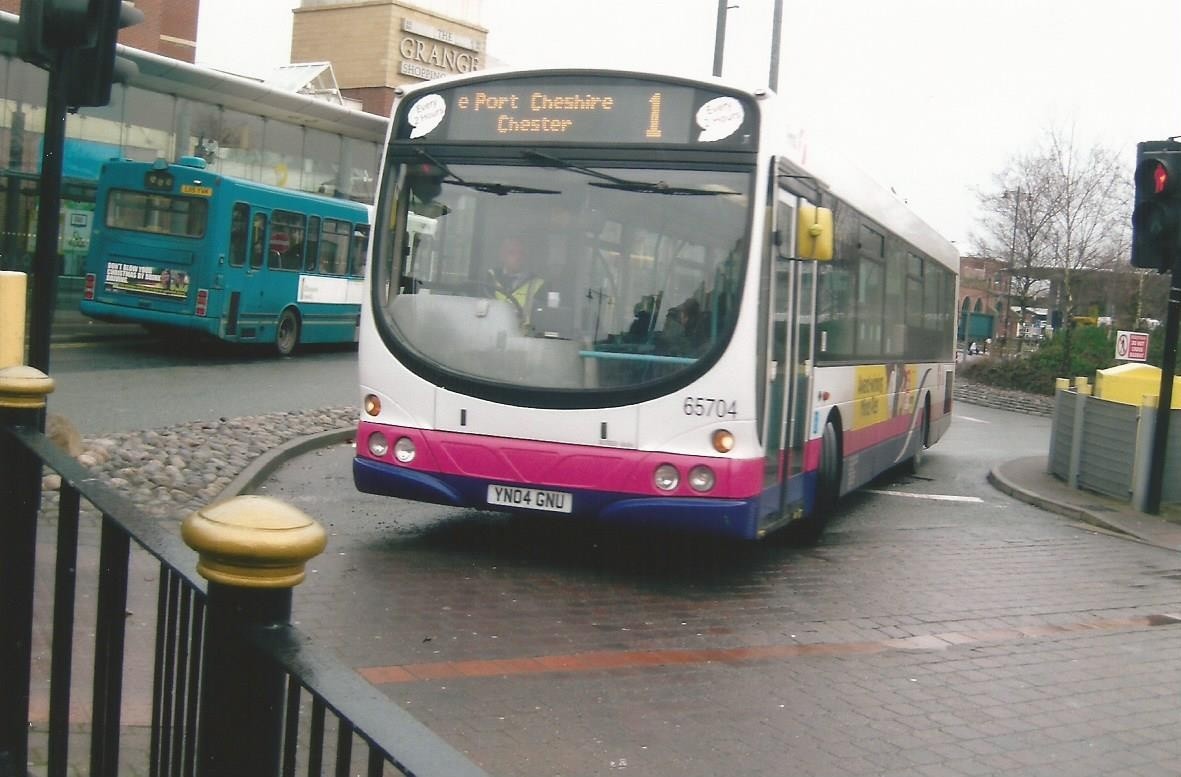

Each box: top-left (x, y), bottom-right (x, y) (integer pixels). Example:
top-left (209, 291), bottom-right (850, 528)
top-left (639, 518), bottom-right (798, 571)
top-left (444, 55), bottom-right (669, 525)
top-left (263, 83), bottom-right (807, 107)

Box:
top-left (998, 185), bottom-right (1034, 337)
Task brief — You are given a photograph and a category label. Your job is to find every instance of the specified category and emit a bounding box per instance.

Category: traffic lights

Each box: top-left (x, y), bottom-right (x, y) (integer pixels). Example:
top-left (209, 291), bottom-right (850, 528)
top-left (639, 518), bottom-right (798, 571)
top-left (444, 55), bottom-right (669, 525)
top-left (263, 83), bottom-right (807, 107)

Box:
top-left (1131, 140), bottom-right (1181, 268)
top-left (65, 0), bottom-right (145, 109)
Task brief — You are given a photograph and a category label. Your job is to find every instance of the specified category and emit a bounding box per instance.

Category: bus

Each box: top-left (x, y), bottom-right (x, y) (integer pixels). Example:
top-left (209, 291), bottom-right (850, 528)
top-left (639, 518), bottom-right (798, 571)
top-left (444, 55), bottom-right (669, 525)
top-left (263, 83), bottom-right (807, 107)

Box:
top-left (351, 65), bottom-right (961, 550)
top-left (75, 154), bottom-right (439, 360)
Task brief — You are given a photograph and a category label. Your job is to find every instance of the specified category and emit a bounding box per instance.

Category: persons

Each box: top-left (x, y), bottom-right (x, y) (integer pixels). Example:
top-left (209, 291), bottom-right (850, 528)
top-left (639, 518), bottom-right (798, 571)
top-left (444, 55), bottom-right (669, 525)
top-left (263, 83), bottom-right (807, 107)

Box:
top-left (457, 235), bottom-right (548, 339)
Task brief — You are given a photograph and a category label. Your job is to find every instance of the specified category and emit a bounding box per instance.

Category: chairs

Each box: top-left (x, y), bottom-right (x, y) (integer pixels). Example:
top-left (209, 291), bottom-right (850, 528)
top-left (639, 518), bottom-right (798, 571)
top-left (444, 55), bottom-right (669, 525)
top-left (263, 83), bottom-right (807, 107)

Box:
top-left (269, 248), bottom-right (313, 270)
